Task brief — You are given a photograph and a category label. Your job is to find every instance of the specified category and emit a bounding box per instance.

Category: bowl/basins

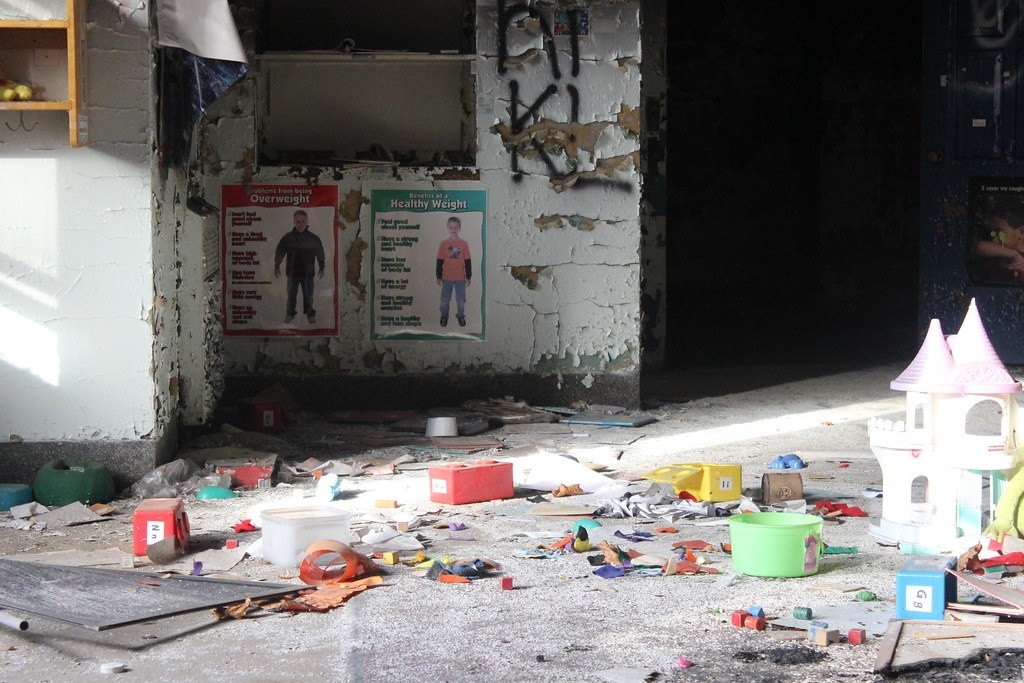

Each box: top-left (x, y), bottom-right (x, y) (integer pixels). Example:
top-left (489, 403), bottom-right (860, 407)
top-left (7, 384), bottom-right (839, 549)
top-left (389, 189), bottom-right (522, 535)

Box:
top-left (729, 512), bottom-right (823, 577)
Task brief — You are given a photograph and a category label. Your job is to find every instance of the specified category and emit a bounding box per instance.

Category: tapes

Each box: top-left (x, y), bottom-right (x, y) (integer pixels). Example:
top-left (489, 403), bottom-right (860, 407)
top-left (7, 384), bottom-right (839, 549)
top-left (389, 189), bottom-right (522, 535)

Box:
top-left (426, 417), bottom-right (458, 437)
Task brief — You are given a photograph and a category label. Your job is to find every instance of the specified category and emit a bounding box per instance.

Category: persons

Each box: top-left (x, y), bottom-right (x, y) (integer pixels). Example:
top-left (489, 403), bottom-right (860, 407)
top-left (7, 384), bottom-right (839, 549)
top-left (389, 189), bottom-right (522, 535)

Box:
top-left (274, 209), bottom-right (326, 323)
top-left (436, 217), bottom-right (473, 326)
top-left (972, 204), bottom-right (1024, 283)
